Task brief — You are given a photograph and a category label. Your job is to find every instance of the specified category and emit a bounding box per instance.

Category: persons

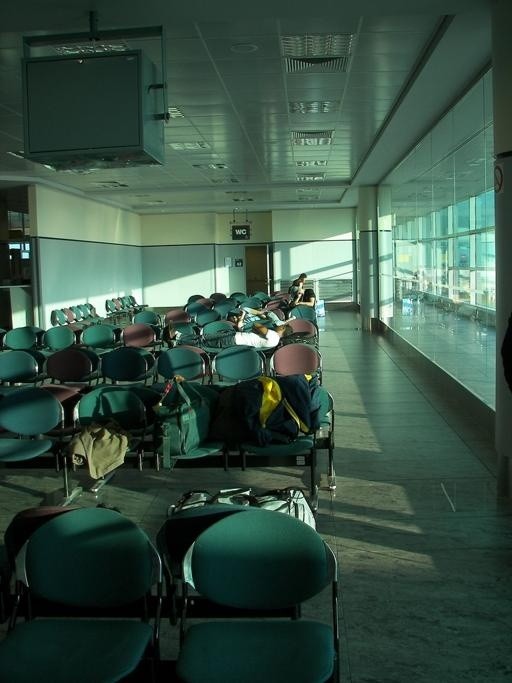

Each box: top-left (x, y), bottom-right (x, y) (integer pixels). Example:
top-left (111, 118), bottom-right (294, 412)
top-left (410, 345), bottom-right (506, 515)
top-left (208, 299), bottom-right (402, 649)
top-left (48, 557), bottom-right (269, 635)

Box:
top-left (168, 321), bottom-right (293, 351)
top-left (227, 307), bottom-right (295, 328)
top-left (290, 286), bottom-right (316, 310)
top-left (289, 273), bottom-right (307, 294)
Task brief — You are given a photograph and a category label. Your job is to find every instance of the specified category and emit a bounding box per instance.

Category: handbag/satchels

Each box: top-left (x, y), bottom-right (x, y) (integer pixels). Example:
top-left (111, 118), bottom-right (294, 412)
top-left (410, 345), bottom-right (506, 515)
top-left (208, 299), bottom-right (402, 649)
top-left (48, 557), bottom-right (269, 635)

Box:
top-left (150, 376), bottom-right (210, 455)
top-left (168, 487), bottom-right (316, 532)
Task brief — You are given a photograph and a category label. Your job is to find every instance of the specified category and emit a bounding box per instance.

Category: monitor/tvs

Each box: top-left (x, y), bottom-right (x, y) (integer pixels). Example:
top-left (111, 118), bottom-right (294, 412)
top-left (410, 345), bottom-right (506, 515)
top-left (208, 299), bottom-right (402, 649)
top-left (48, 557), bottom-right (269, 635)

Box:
top-left (23, 49), bottom-right (168, 167)
